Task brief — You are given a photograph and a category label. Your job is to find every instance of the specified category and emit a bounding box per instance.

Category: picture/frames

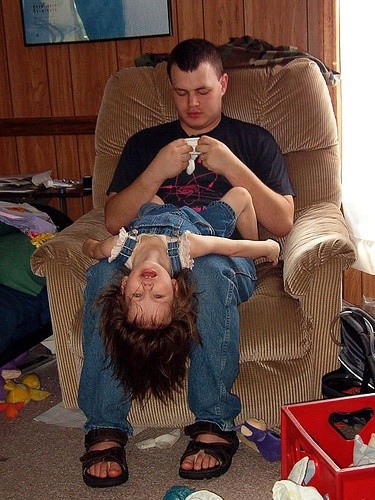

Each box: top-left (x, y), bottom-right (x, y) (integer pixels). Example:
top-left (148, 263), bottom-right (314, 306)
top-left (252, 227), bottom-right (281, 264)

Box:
top-left (20, 0), bottom-right (173, 48)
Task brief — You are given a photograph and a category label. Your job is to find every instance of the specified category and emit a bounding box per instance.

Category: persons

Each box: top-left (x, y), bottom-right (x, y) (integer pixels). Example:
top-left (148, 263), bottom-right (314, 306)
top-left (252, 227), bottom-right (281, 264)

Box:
top-left (81, 186), bottom-right (281, 405)
top-left (77, 38), bottom-right (298, 488)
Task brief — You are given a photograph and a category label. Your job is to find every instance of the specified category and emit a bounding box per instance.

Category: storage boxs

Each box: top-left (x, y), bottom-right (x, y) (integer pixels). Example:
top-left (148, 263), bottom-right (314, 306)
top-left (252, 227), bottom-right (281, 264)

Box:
top-left (281, 393), bottom-right (375, 500)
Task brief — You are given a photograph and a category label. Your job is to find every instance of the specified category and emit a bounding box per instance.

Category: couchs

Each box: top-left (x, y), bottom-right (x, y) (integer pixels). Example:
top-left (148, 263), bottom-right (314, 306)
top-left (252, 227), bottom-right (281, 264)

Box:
top-left (0, 203), bottom-right (75, 368)
top-left (29, 56), bottom-right (356, 430)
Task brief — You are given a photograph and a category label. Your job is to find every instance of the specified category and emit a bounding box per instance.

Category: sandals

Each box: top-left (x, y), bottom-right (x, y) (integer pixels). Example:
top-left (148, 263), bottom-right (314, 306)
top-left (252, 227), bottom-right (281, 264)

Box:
top-left (178, 420), bottom-right (240, 480)
top-left (79, 428), bottom-right (129, 489)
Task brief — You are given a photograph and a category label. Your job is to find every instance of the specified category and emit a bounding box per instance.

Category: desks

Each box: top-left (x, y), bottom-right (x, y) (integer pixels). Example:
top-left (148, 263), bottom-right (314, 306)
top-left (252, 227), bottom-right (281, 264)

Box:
top-left (0, 180), bottom-right (92, 217)
